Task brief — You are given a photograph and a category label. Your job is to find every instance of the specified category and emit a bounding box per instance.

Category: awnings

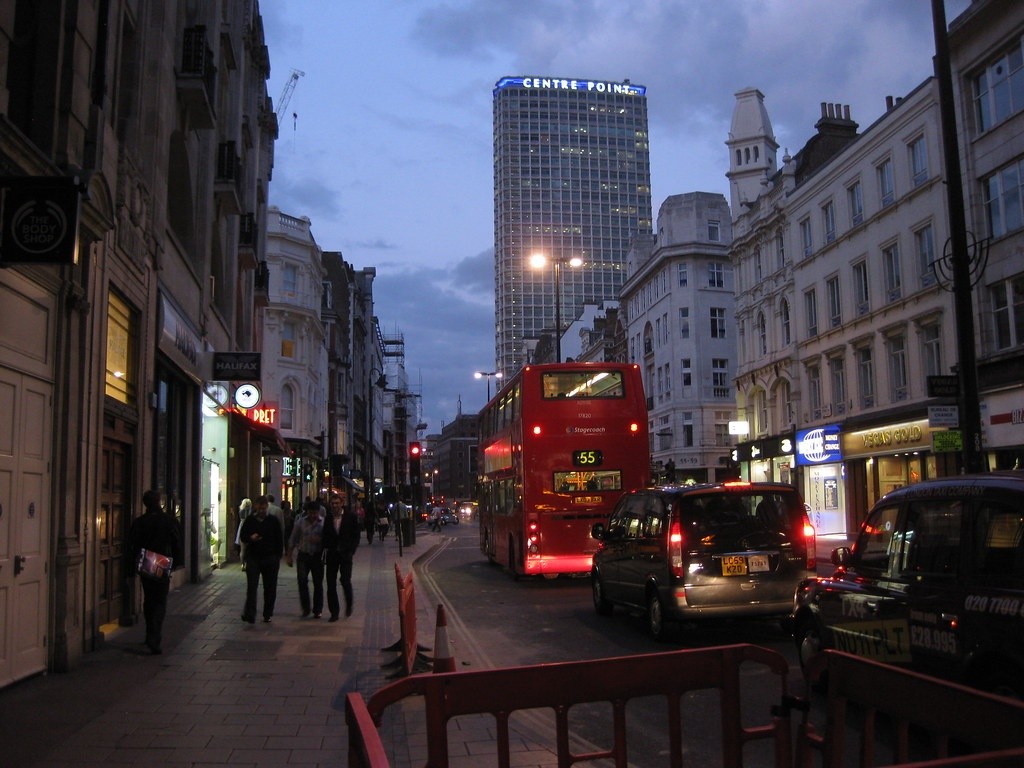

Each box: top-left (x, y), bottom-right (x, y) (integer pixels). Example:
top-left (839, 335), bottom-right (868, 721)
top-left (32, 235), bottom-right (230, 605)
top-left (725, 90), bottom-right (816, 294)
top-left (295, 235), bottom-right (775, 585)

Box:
top-left (344, 476), bottom-right (364, 492)
top-left (235, 411), bottom-right (294, 458)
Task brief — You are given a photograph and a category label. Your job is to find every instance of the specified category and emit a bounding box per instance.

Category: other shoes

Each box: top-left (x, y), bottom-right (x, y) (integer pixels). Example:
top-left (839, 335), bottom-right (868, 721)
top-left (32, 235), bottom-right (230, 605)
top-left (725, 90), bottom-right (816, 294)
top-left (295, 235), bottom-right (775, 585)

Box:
top-left (303, 610), bottom-right (310, 617)
top-left (265, 615), bottom-right (271, 623)
top-left (241, 562), bottom-right (247, 571)
top-left (347, 601), bottom-right (353, 617)
top-left (330, 613), bottom-right (338, 621)
top-left (144, 635), bottom-right (162, 654)
top-left (241, 613), bottom-right (255, 624)
top-left (314, 611), bottom-right (322, 617)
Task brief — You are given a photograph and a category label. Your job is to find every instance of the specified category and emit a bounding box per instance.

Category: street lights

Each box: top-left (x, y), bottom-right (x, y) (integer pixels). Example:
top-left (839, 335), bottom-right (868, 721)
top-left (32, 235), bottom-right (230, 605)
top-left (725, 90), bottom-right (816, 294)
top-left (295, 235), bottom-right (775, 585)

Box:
top-left (475, 371), bottom-right (503, 403)
top-left (532, 256), bottom-right (582, 362)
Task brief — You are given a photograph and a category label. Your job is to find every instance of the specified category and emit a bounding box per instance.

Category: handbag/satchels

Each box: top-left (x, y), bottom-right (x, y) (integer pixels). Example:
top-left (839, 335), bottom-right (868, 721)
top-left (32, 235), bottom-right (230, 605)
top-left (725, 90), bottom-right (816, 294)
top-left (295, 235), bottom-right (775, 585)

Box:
top-left (134, 548), bottom-right (173, 584)
top-left (379, 518), bottom-right (388, 526)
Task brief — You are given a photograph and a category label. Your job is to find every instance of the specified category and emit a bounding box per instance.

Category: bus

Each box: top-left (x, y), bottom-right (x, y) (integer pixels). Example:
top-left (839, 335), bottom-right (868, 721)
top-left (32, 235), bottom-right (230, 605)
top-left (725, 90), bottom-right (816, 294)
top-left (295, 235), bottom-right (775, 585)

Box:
top-left (474, 361), bottom-right (652, 583)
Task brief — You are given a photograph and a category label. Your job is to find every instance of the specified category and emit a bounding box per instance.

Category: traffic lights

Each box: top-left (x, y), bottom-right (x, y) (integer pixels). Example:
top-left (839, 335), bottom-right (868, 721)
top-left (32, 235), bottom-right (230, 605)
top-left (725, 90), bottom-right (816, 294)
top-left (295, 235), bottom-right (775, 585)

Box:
top-left (410, 441), bottom-right (422, 476)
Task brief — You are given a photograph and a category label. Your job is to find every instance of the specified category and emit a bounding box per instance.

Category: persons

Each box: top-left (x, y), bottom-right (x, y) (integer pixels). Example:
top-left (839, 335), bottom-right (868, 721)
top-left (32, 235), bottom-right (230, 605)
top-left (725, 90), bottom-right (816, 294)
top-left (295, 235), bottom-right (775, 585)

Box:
top-left (129, 490), bottom-right (180, 655)
top-left (432, 504), bottom-right (442, 532)
top-left (757, 495), bottom-right (786, 520)
top-left (235, 493), bottom-right (410, 624)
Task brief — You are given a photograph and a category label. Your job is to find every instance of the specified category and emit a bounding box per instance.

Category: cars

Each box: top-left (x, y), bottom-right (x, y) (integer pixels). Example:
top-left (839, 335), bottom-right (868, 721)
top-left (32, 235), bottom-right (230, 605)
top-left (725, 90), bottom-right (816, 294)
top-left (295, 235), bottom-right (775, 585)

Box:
top-left (428, 508), bottom-right (459, 525)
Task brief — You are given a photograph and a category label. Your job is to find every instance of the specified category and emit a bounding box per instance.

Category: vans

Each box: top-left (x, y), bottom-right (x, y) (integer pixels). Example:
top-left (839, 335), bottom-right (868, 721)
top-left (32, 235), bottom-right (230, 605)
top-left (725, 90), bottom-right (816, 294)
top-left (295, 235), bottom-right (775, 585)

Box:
top-left (591, 481), bottom-right (817, 645)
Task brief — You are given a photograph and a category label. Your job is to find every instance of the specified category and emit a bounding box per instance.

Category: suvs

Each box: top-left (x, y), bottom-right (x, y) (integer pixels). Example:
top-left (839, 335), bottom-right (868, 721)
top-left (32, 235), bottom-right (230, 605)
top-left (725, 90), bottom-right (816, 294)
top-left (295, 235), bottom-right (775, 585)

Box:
top-left (790, 469), bottom-right (1024, 744)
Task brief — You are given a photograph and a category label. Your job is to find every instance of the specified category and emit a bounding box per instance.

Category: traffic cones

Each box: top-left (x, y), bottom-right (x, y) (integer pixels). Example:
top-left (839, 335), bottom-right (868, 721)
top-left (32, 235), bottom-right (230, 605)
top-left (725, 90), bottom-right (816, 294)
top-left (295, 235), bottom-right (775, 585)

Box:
top-left (427, 602), bottom-right (460, 675)
